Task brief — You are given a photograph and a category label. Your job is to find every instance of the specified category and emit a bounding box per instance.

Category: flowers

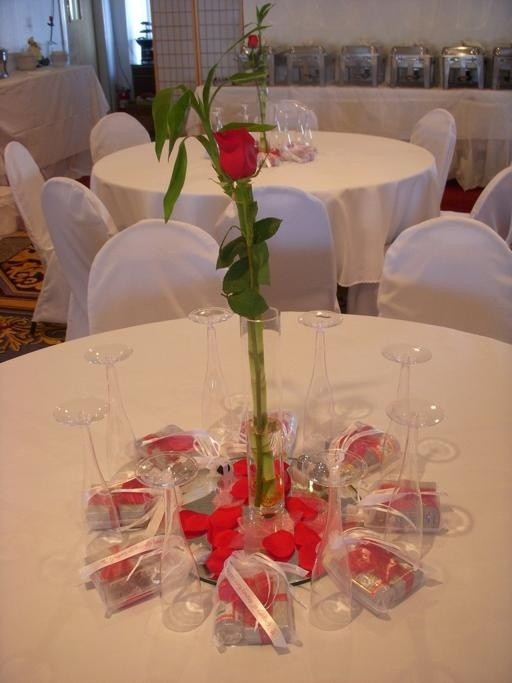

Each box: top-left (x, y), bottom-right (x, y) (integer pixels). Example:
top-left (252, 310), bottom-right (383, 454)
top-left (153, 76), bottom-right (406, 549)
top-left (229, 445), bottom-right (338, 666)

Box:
top-left (150, 40), bottom-right (305, 505)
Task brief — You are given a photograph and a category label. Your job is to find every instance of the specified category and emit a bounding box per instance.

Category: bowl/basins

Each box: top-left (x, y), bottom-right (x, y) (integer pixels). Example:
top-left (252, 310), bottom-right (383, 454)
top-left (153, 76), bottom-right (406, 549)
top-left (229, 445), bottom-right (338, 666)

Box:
top-left (13, 52), bottom-right (39, 71)
top-left (49, 51), bottom-right (68, 67)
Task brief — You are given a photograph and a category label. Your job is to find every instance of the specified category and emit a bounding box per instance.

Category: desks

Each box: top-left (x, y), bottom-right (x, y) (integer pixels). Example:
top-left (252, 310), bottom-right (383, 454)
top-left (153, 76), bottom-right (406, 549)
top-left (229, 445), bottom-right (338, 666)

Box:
top-left (189, 73), bottom-right (512, 188)
top-left (0, 58), bottom-right (110, 186)
top-left (85, 127), bottom-right (437, 287)
top-left (0, 310), bottom-right (512, 683)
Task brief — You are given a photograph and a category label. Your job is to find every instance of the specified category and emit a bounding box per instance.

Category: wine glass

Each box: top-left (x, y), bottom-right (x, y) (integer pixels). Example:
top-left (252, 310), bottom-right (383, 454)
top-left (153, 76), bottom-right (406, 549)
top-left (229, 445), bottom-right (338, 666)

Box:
top-left (187, 307), bottom-right (233, 439)
top-left (84, 341), bottom-right (138, 477)
top-left (301, 449), bottom-right (370, 631)
top-left (385, 396), bottom-right (445, 559)
top-left (132, 450), bottom-right (206, 632)
top-left (48, 396), bottom-right (124, 560)
top-left (201, 99), bottom-right (320, 174)
top-left (378, 341), bottom-right (433, 481)
top-left (296, 309), bottom-right (343, 448)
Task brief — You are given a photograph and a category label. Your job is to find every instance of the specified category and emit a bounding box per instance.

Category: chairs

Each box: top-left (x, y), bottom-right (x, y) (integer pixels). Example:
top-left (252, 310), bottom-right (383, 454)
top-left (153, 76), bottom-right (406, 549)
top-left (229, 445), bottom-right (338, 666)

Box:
top-left (408, 105), bottom-right (460, 210)
top-left (29, 176), bottom-right (118, 337)
top-left (262, 99), bottom-right (322, 131)
top-left (88, 111), bottom-right (153, 165)
top-left (87, 217), bottom-right (230, 338)
top-left (237, 183), bottom-right (344, 316)
top-left (2, 140), bottom-right (55, 268)
top-left (375, 164), bottom-right (512, 339)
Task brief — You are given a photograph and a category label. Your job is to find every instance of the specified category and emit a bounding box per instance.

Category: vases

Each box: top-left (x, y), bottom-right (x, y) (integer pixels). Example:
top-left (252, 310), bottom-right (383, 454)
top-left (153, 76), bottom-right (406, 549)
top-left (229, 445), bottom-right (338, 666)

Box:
top-left (232, 304), bottom-right (291, 520)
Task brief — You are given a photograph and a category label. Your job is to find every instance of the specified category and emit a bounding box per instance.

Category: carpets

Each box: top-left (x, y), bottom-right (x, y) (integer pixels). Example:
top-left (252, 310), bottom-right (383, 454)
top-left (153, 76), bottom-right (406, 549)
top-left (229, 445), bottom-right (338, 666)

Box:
top-left (2, 228), bottom-right (73, 366)
